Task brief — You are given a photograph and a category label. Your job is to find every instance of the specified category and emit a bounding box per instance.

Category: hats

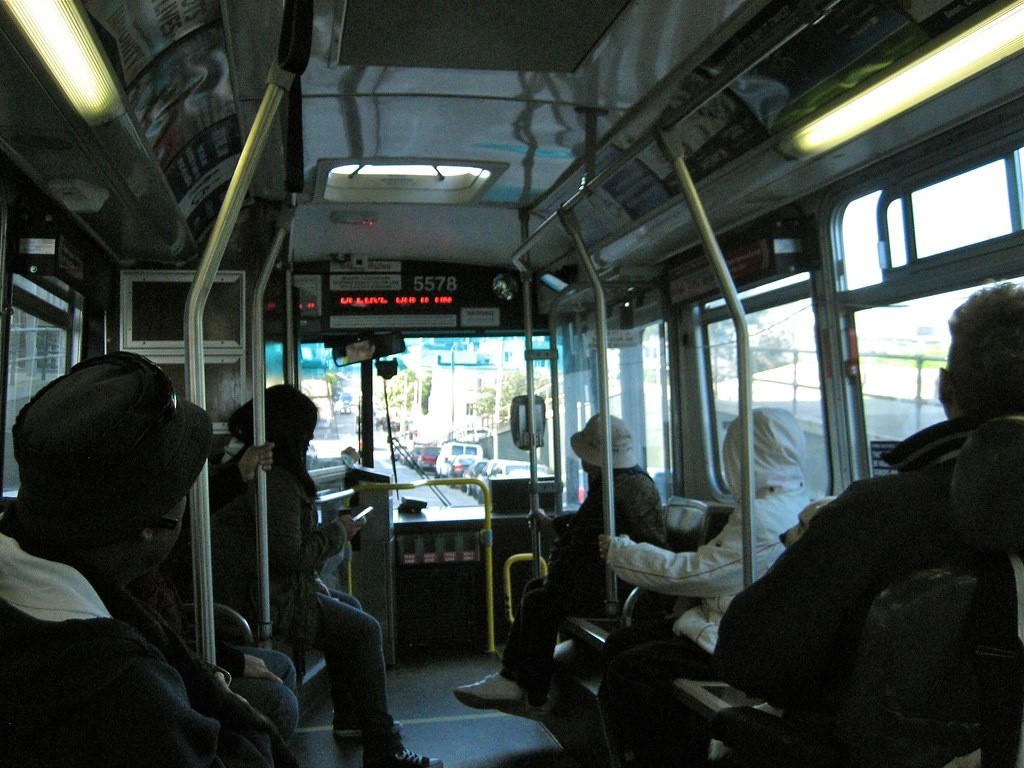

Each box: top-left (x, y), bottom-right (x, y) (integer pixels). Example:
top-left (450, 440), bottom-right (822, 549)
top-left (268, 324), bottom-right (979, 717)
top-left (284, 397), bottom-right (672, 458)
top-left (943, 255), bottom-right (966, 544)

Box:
top-left (3, 354), bottom-right (213, 539)
top-left (570, 413), bottom-right (638, 469)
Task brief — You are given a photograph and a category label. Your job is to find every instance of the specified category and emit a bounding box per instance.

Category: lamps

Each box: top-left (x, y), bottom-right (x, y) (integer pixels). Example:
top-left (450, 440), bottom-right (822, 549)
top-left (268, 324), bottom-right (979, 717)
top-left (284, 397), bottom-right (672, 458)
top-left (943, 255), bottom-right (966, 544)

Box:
top-left (0, 0), bottom-right (129, 130)
top-left (771, 0), bottom-right (1024, 171)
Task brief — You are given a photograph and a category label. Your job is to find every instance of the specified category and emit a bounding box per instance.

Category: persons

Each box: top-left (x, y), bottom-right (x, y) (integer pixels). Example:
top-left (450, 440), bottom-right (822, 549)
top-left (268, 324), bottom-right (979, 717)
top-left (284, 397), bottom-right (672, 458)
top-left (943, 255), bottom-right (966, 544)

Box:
top-left (126, 442), bottom-right (299, 745)
top-left (453, 414), bottom-right (671, 722)
top-left (336, 340), bottom-right (375, 366)
top-left (211, 385), bottom-right (444, 768)
top-left (713, 281), bottom-right (1024, 709)
top-left (0, 351), bottom-right (297, 768)
top-left (305, 447), bottom-right (360, 470)
top-left (595, 408), bottom-right (810, 768)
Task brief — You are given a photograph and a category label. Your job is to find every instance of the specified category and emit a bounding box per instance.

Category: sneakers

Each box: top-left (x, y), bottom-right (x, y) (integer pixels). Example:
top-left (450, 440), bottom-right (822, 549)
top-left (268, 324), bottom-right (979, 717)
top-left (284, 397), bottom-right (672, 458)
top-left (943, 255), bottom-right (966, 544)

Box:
top-left (498, 698), bottom-right (554, 721)
top-left (334, 719), bottom-right (404, 738)
top-left (361, 744), bottom-right (444, 768)
top-left (453, 673), bottom-right (527, 710)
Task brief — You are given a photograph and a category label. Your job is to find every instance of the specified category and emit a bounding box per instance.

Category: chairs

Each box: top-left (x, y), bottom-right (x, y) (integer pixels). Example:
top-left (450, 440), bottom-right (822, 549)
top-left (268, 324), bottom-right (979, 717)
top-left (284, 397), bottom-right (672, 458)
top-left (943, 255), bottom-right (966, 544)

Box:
top-left (550, 414), bottom-right (1024, 768)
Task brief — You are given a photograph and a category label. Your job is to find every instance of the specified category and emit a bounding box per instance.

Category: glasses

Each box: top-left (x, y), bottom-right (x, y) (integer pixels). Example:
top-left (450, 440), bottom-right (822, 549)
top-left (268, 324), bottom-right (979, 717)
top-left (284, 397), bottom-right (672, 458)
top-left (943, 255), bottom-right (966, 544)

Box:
top-left (119, 354), bottom-right (179, 463)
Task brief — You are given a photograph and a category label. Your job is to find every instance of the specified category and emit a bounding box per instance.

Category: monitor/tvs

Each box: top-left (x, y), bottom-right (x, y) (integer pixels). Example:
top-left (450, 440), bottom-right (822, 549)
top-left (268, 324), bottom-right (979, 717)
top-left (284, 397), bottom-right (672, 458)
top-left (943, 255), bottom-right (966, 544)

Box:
top-left (120, 270), bottom-right (246, 364)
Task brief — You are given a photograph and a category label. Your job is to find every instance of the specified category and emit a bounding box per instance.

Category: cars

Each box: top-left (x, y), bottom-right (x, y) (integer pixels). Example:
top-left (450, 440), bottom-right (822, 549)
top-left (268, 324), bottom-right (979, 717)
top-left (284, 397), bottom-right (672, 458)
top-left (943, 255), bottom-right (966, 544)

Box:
top-left (391, 440), bottom-right (556, 505)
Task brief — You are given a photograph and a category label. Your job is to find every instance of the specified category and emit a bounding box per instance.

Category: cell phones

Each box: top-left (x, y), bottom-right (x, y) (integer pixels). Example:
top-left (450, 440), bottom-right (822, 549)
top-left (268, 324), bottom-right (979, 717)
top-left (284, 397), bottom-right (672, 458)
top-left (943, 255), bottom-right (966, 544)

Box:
top-left (352, 506), bottom-right (374, 522)
